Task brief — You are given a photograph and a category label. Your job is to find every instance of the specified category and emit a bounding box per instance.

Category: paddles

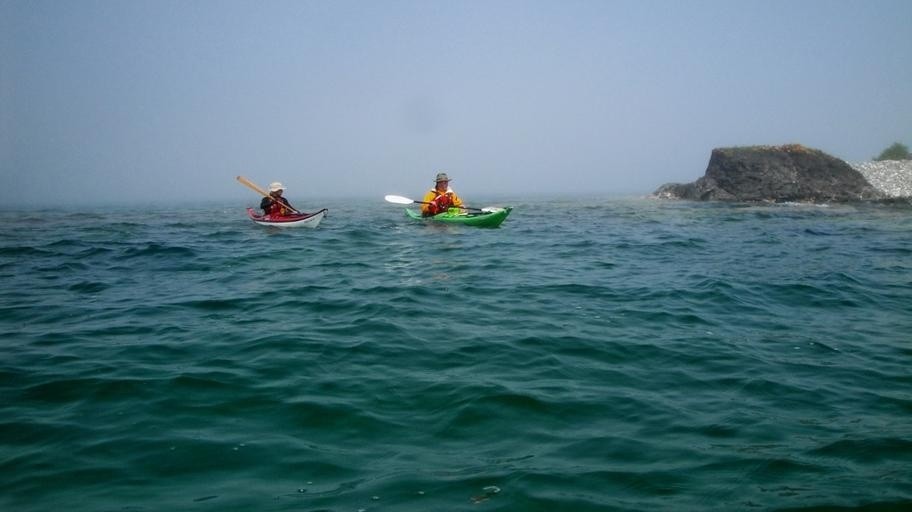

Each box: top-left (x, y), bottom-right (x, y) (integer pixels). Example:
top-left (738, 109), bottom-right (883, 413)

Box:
top-left (237, 176), bottom-right (300, 214)
top-left (385, 195), bottom-right (504, 213)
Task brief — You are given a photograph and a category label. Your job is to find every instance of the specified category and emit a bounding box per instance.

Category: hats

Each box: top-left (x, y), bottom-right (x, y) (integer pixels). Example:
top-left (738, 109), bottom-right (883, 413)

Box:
top-left (269, 182), bottom-right (285, 192)
top-left (433, 173), bottom-right (451, 183)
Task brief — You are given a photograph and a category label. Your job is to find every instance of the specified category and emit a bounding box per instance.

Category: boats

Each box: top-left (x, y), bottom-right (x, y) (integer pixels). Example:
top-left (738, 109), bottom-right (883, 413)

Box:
top-left (245, 207), bottom-right (328, 231)
top-left (407, 206), bottom-right (514, 229)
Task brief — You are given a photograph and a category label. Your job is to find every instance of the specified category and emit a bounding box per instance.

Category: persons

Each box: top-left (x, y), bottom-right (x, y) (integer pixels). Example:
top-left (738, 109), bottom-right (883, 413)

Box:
top-left (259, 183), bottom-right (300, 217)
top-left (421, 173), bottom-right (465, 217)
